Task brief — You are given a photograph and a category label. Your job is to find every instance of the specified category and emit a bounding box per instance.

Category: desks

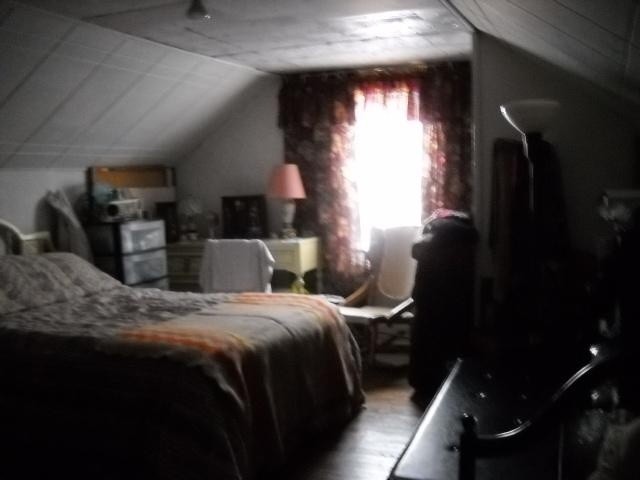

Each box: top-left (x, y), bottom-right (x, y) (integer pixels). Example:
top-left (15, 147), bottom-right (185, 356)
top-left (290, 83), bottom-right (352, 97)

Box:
top-left (387, 350), bottom-right (640, 480)
top-left (165, 239), bottom-right (323, 294)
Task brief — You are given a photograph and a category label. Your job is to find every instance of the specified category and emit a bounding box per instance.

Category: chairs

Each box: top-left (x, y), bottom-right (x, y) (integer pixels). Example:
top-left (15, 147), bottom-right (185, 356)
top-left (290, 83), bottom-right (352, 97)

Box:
top-left (198, 237), bottom-right (276, 294)
top-left (337, 224), bottom-right (425, 366)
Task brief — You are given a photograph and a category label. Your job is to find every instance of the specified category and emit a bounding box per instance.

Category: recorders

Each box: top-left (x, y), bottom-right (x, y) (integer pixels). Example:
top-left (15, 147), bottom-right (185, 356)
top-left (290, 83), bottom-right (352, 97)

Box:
top-left (100, 199), bottom-right (144, 224)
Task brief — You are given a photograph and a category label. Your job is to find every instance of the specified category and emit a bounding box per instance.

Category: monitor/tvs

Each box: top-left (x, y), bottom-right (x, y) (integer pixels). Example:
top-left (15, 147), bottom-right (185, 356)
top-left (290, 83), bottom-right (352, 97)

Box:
top-left (222, 195), bottom-right (268, 239)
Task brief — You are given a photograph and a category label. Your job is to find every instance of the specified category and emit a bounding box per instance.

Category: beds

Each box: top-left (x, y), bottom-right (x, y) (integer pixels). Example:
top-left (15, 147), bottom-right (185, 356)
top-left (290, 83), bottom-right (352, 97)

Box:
top-left (0, 219), bottom-right (347, 480)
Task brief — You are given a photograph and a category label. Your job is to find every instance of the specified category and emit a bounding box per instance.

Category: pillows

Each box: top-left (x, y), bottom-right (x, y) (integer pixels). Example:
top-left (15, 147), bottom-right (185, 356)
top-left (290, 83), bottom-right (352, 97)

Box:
top-left (0, 251), bottom-right (123, 315)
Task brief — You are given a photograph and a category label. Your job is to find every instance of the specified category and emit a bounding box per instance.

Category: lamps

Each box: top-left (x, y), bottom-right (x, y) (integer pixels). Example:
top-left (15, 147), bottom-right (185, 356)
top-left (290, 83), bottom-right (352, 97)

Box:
top-left (265, 163), bottom-right (307, 238)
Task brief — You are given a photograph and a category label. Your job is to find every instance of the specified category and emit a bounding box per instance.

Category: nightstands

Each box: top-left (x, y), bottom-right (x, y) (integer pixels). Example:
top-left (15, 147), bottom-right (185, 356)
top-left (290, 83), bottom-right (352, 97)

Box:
top-left (82, 217), bottom-right (171, 292)
